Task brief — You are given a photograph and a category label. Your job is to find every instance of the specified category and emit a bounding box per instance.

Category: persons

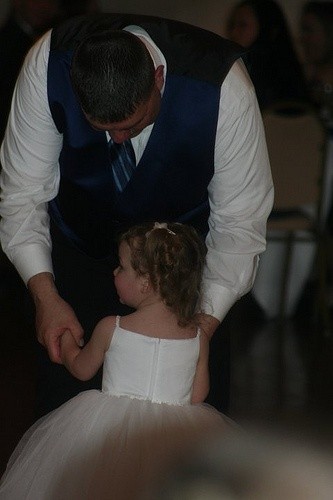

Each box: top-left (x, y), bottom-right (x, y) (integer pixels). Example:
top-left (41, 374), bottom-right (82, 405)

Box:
top-left (227, 1), bottom-right (332, 369)
top-left (53, 218), bottom-right (210, 500)
top-left (0, 12), bottom-right (276, 425)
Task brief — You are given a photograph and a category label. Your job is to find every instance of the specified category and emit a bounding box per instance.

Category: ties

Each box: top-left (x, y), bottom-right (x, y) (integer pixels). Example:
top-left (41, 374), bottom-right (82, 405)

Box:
top-left (108, 137), bottom-right (137, 197)
